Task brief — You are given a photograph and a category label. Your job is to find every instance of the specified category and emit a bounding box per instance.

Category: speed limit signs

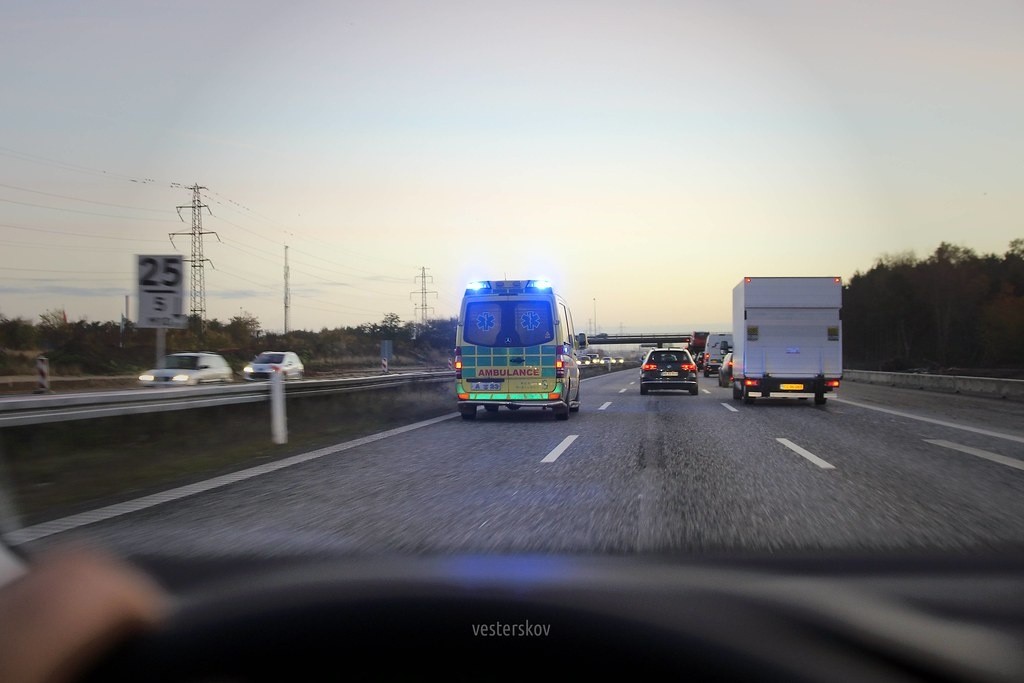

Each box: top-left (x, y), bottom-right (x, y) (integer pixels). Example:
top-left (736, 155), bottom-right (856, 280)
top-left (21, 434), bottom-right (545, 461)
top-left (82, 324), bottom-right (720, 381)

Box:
top-left (136, 254), bottom-right (186, 331)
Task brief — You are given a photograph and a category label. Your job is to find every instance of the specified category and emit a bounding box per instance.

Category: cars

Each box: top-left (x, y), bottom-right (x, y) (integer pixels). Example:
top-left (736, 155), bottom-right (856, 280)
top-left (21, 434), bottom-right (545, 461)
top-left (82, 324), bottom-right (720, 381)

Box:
top-left (685, 331), bottom-right (709, 372)
top-left (716, 350), bottom-right (733, 387)
top-left (243, 351), bottom-right (305, 382)
top-left (138, 352), bottom-right (235, 391)
top-left (580, 354), bottom-right (625, 366)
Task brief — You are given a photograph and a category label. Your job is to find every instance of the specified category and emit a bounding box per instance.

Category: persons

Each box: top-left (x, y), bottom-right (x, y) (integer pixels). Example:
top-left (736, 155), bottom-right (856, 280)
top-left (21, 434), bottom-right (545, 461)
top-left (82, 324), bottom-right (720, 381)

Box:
top-left (0, 548), bottom-right (170, 683)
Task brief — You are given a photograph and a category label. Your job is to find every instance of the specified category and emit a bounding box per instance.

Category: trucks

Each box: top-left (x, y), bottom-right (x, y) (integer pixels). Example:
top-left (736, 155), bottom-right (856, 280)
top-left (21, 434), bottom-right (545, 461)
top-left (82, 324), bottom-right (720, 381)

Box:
top-left (720, 277), bottom-right (843, 405)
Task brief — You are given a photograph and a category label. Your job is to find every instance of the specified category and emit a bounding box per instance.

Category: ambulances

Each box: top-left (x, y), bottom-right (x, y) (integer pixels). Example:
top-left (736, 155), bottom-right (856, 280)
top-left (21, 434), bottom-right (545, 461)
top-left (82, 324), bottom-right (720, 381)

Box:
top-left (454, 279), bottom-right (588, 420)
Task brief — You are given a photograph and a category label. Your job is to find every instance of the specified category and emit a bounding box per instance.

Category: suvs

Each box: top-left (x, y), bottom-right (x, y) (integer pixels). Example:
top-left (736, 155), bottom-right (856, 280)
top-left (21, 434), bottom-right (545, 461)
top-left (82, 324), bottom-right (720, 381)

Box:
top-left (638, 346), bottom-right (700, 395)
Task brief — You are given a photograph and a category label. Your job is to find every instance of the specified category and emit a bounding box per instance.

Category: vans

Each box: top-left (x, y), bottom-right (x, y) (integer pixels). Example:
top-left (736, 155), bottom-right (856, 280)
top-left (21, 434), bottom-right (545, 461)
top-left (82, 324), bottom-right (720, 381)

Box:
top-left (704, 333), bottom-right (734, 378)
